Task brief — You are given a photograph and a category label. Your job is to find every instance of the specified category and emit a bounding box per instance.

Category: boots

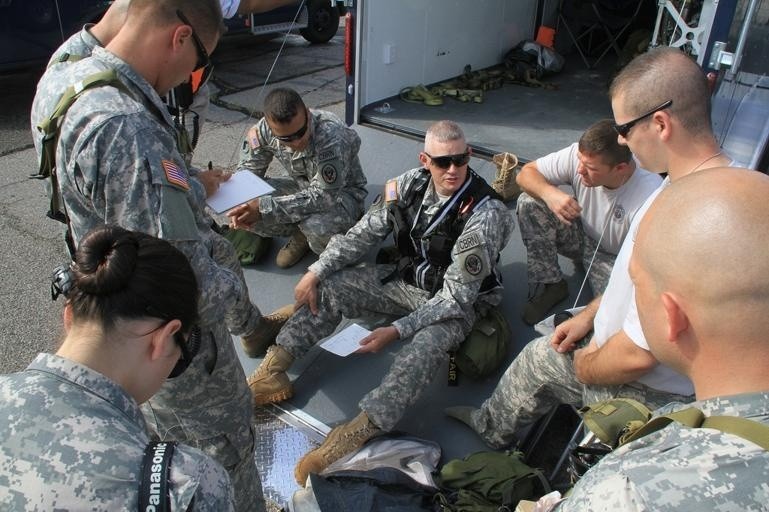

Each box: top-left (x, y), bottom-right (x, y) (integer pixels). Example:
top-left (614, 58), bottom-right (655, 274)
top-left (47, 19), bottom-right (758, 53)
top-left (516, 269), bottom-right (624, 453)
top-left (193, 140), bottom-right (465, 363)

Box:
top-left (491, 152), bottom-right (523, 201)
top-left (277, 233), bottom-right (309, 267)
top-left (241, 303), bottom-right (295, 358)
top-left (295, 413), bottom-right (383, 487)
top-left (247, 346), bottom-right (295, 406)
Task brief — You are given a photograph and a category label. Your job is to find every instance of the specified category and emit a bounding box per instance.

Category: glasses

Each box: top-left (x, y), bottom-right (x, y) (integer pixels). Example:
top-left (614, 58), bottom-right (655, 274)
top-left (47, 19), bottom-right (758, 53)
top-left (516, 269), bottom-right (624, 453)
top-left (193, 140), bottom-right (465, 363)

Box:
top-left (613, 100), bottom-right (671, 138)
top-left (148, 311), bottom-right (192, 376)
top-left (424, 149), bottom-right (469, 170)
top-left (174, 8), bottom-right (213, 71)
top-left (273, 109), bottom-right (308, 143)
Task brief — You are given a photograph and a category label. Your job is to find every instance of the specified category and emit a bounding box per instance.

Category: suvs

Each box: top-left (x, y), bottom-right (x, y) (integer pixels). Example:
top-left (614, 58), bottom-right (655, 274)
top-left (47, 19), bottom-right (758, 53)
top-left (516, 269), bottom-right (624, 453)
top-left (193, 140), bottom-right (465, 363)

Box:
top-left (220, 1), bottom-right (344, 50)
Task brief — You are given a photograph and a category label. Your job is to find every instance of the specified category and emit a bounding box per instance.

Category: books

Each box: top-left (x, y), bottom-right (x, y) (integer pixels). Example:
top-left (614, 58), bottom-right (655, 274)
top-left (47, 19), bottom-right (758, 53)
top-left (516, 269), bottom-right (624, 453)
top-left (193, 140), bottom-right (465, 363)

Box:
top-left (205, 169), bottom-right (276, 215)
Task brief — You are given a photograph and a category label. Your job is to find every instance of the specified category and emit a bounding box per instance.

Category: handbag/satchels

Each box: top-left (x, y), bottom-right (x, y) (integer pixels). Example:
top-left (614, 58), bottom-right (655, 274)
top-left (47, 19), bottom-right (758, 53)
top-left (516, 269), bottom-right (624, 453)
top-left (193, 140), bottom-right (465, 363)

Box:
top-left (224, 229), bottom-right (272, 266)
top-left (447, 302), bottom-right (512, 385)
top-left (503, 40), bottom-right (566, 90)
top-left (283, 431), bottom-right (444, 512)
top-left (568, 398), bottom-right (650, 482)
top-left (432, 449), bottom-right (550, 511)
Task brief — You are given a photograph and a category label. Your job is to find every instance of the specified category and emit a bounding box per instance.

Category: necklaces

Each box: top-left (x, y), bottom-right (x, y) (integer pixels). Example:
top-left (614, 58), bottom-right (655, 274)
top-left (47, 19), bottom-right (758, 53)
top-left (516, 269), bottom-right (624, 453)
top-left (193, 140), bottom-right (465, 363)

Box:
top-left (687, 151), bottom-right (725, 174)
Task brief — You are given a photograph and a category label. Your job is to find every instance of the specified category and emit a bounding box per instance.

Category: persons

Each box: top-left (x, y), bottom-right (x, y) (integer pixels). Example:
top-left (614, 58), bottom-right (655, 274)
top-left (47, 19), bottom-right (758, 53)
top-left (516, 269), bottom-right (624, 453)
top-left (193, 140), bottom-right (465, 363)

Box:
top-left (160, 79), bottom-right (209, 154)
top-left (514, 118), bottom-right (666, 326)
top-left (224, 88), bottom-right (369, 270)
top-left (47, 1), bottom-right (297, 359)
top-left (510, 165), bottom-right (767, 512)
top-left (0, 226), bottom-right (236, 510)
top-left (442, 46), bottom-right (747, 471)
top-left (29, 1), bottom-right (265, 511)
top-left (246, 119), bottom-right (515, 489)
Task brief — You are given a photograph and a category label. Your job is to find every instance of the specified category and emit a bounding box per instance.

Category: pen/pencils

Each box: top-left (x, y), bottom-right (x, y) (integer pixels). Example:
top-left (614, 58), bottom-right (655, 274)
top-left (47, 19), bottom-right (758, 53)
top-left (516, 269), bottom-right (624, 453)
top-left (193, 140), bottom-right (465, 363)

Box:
top-left (207, 161), bottom-right (213, 170)
